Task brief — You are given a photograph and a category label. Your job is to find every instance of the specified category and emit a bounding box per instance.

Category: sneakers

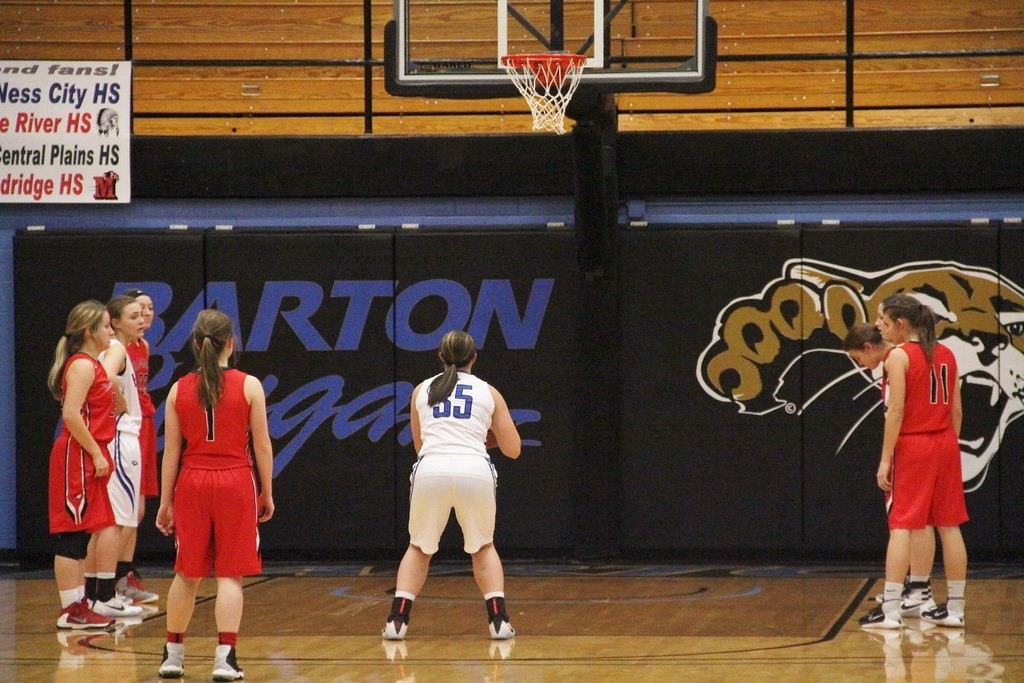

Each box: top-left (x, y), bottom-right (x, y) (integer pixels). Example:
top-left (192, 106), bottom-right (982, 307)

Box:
top-left (158, 642), bottom-right (185, 677)
top-left (921, 598), bottom-right (966, 627)
top-left (488, 615), bottom-right (515, 639)
top-left (55, 597), bottom-right (115, 629)
top-left (382, 615), bottom-right (409, 640)
top-left (868, 585), bottom-right (909, 602)
top-left (860, 599), bottom-right (904, 628)
top-left (86, 598), bottom-right (142, 616)
top-left (115, 590), bottom-right (134, 606)
top-left (899, 583), bottom-right (938, 618)
top-left (126, 572), bottom-right (144, 591)
top-left (115, 576), bottom-right (160, 603)
top-left (212, 645), bottom-right (243, 681)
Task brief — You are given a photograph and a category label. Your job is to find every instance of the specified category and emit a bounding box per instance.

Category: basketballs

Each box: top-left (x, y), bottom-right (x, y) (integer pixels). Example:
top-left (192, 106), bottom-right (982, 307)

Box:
top-left (485, 428), bottom-right (499, 450)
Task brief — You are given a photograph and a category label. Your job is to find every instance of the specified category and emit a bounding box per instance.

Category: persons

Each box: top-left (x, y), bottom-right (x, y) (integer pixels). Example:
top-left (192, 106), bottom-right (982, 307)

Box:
top-left (155, 308), bottom-right (276, 680)
top-left (380, 331), bottom-right (521, 640)
top-left (859, 298), bottom-right (969, 629)
top-left (875, 295), bottom-right (938, 620)
top-left (85, 295), bottom-right (145, 618)
top-left (843, 323), bottom-right (911, 605)
top-left (118, 287), bottom-right (160, 605)
top-left (47, 300), bottom-right (116, 632)
